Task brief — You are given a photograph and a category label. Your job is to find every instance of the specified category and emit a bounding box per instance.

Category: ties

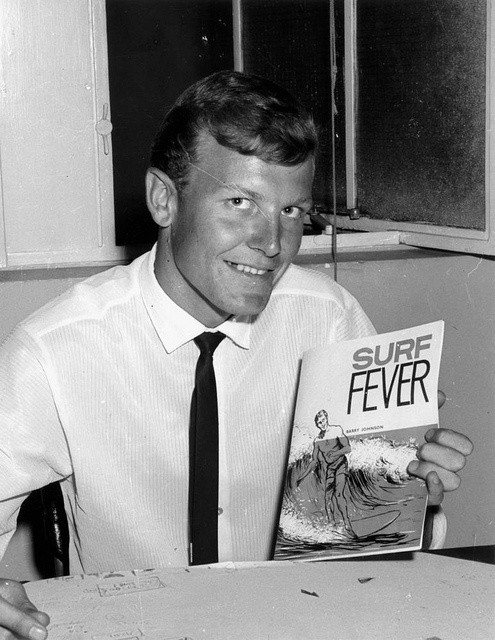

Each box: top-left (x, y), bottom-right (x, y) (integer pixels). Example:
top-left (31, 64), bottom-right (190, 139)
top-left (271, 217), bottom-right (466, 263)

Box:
top-left (189, 332), bottom-right (227, 566)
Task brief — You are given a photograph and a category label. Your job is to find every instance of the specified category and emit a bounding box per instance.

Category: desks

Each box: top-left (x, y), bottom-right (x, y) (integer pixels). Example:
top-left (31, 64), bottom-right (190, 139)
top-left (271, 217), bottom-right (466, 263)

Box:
top-left (23, 550), bottom-right (495, 640)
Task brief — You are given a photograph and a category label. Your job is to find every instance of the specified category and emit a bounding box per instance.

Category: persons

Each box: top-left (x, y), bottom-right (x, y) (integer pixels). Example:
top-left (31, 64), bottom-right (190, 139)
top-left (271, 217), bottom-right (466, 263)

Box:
top-left (0, 71), bottom-right (476, 640)
top-left (310, 408), bottom-right (360, 541)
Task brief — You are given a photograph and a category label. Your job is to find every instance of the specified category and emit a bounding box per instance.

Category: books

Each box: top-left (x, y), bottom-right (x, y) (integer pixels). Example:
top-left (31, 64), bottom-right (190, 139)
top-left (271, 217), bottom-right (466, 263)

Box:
top-left (274, 319), bottom-right (444, 561)
